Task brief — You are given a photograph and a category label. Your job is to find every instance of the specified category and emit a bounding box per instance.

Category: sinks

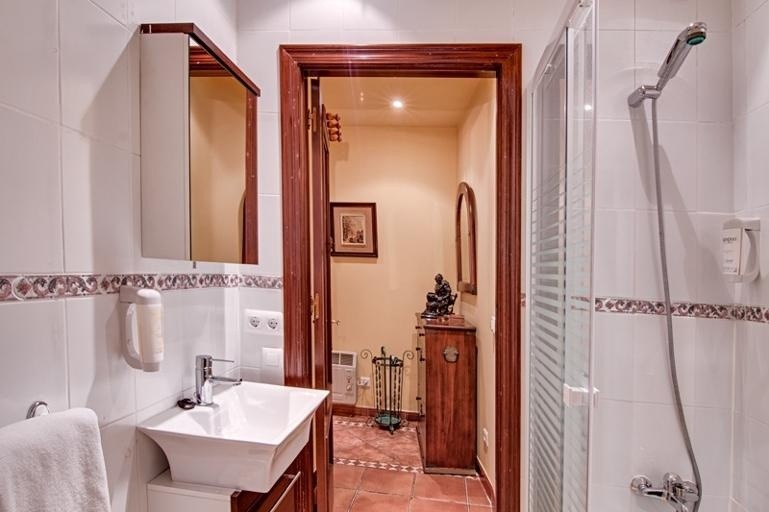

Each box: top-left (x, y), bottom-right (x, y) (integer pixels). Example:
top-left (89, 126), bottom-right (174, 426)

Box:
top-left (136, 380), bottom-right (330, 494)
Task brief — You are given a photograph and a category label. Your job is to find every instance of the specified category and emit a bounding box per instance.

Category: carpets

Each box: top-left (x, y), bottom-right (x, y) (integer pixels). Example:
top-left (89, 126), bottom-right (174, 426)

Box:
top-left (332, 414), bottom-right (481, 481)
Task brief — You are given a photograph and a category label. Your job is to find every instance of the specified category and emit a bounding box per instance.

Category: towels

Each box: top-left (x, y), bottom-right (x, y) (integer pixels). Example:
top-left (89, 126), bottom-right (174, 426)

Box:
top-left (0, 408), bottom-right (111, 512)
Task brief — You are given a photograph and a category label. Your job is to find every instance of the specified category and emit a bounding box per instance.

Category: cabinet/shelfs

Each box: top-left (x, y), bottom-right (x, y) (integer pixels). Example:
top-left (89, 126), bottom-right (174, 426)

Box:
top-left (144, 464), bottom-right (301, 512)
top-left (415, 311), bottom-right (477, 475)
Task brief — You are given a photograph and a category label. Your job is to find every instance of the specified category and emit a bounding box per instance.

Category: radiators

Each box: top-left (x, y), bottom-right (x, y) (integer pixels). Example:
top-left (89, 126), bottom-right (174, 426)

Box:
top-left (332, 351), bottom-right (358, 405)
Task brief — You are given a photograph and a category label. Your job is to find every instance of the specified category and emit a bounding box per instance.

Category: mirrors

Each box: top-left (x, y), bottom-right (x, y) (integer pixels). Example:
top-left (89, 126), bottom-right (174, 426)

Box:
top-left (188, 34), bottom-right (260, 265)
top-left (454, 181), bottom-right (479, 296)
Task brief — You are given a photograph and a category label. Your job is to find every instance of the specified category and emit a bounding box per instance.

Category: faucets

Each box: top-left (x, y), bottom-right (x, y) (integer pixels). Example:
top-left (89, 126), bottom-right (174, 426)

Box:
top-left (194, 355), bottom-right (243, 406)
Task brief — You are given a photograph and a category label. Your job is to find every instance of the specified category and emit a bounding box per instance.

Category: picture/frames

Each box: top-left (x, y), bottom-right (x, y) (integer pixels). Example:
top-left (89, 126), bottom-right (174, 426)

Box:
top-left (330, 201), bottom-right (379, 259)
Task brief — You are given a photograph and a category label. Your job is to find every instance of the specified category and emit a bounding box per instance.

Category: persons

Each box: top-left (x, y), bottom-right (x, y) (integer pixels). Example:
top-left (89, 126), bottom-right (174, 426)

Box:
top-left (425, 272), bottom-right (454, 317)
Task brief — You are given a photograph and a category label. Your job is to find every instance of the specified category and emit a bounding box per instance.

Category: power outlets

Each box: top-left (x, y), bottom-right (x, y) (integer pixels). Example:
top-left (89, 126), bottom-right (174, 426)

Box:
top-left (360, 377), bottom-right (370, 388)
top-left (243, 309), bottom-right (283, 337)
